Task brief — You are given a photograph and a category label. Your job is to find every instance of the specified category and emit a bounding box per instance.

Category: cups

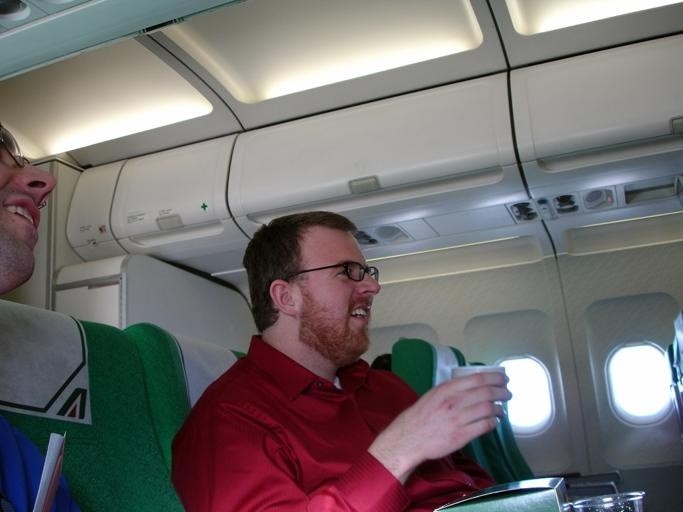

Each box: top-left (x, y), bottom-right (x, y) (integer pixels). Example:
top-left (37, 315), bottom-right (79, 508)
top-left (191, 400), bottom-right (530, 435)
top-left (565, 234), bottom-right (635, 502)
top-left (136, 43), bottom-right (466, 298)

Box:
top-left (451, 365), bottom-right (505, 408)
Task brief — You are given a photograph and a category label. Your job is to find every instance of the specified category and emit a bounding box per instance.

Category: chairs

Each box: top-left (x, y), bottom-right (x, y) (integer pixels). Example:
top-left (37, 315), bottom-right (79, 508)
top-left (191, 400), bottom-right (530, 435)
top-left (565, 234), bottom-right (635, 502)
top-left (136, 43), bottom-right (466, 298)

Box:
top-left (0, 298), bottom-right (539, 511)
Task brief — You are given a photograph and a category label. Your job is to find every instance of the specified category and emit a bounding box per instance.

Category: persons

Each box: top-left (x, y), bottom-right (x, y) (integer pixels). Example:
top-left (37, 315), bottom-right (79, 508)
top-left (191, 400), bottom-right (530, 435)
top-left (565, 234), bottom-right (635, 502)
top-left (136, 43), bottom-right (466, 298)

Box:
top-left (0, 123), bottom-right (84, 512)
top-left (166, 207), bottom-right (515, 510)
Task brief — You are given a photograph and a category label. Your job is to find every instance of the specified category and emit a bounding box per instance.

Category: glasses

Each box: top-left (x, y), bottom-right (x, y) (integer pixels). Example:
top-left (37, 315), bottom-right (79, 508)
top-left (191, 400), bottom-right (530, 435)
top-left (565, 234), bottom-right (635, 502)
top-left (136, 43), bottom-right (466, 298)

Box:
top-left (286, 262), bottom-right (378, 282)
top-left (0, 128), bottom-right (46, 209)
